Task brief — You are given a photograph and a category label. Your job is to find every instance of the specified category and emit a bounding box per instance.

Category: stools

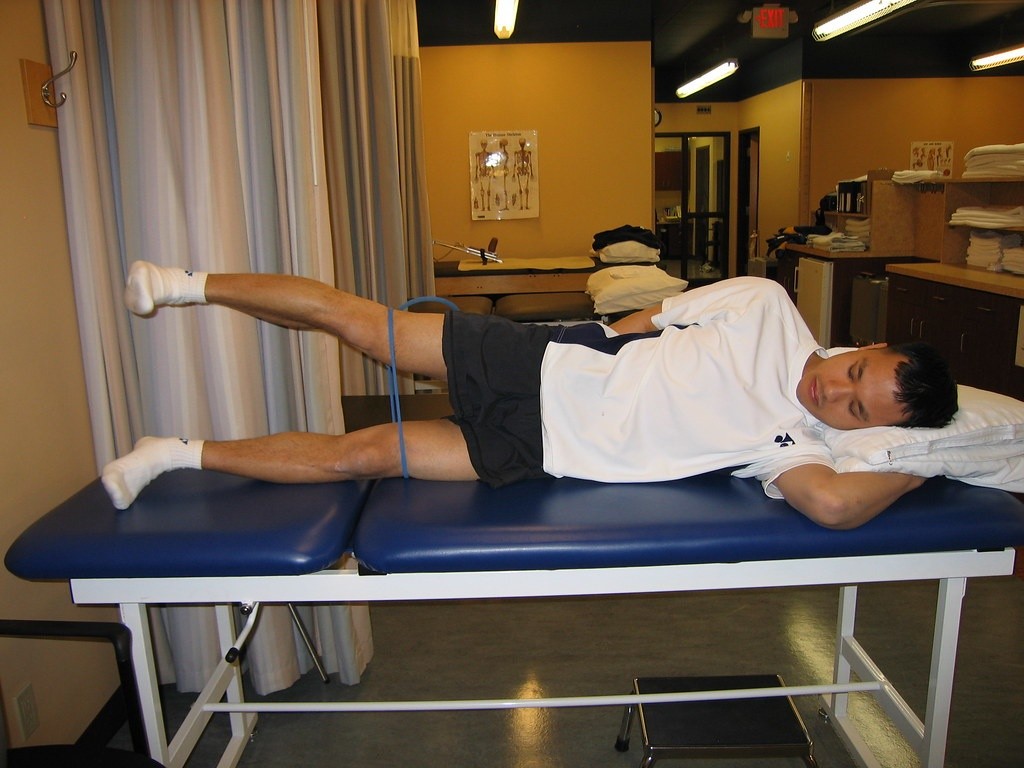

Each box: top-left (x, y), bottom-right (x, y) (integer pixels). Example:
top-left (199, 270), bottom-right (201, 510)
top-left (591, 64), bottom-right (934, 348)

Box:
top-left (615, 674), bottom-right (822, 768)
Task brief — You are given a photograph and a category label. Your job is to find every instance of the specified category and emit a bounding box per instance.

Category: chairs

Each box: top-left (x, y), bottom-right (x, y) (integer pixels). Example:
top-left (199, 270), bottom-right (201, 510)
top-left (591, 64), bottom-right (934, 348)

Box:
top-left (0, 618), bottom-right (165, 768)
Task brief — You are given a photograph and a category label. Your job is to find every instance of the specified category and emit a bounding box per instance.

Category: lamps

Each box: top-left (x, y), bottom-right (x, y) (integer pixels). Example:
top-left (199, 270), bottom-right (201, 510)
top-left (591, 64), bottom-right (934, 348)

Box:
top-left (969, 44), bottom-right (1024, 72)
top-left (676, 58), bottom-right (739, 97)
top-left (736, 7), bottom-right (798, 39)
top-left (812, 0), bottom-right (916, 41)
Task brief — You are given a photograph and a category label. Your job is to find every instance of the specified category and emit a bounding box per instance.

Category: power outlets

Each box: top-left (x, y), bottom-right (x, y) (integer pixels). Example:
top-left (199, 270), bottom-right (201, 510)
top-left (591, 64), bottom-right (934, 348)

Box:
top-left (13, 682), bottom-right (40, 742)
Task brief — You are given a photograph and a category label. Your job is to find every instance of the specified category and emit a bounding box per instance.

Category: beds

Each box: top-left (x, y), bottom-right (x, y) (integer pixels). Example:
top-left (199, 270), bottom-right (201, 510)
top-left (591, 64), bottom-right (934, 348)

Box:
top-left (433, 255), bottom-right (657, 293)
top-left (5, 454), bottom-right (1024, 768)
top-left (401, 292), bottom-right (686, 395)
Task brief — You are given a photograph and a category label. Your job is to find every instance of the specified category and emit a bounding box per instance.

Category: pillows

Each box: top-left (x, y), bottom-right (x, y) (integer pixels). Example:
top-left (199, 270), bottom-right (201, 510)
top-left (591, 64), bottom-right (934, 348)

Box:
top-left (584, 264), bottom-right (689, 315)
top-left (814, 345), bottom-right (1023, 493)
top-left (589, 240), bottom-right (661, 263)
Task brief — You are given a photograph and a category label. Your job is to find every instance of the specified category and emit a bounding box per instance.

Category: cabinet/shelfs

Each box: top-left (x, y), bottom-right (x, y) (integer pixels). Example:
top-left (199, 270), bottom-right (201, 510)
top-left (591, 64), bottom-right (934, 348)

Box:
top-left (810, 169), bottom-right (915, 252)
top-left (784, 249), bottom-right (940, 345)
top-left (941, 177), bottom-right (1024, 278)
top-left (655, 224), bottom-right (694, 259)
top-left (888, 274), bottom-right (1024, 404)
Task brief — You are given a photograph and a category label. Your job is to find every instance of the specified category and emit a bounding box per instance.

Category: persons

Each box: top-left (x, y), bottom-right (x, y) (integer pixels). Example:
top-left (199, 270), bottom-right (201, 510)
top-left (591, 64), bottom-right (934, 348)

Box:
top-left (100, 261), bottom-right (960, 531)
